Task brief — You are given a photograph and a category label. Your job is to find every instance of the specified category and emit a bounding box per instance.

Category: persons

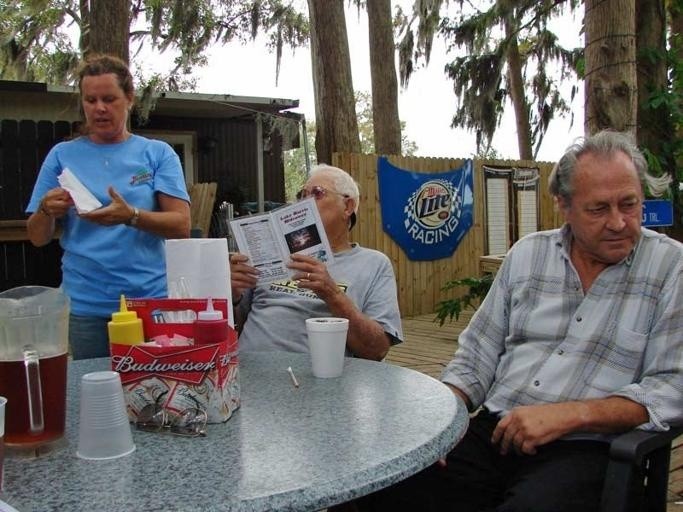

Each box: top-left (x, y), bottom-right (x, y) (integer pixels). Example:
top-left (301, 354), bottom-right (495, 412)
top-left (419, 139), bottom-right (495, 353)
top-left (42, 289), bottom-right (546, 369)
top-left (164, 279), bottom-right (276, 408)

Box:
top-left (225, 164), bottom-right (402, 360)
top-left (23, 55), bottom-right (191, 360)
top-left (328, 129), bottom-right (682, 512)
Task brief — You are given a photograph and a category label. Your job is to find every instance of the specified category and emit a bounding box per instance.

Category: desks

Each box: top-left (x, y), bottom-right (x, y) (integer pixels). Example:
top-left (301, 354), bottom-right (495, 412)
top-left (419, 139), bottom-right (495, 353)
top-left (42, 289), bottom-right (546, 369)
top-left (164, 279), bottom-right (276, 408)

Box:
top-left (1, 343), bottom-right (470, 512)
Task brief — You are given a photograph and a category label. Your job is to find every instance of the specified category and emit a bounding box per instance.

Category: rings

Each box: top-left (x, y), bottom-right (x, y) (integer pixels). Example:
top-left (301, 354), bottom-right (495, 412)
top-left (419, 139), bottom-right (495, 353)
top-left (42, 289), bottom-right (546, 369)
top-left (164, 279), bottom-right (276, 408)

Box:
top-left (306, 272), bottom-right (310, 280)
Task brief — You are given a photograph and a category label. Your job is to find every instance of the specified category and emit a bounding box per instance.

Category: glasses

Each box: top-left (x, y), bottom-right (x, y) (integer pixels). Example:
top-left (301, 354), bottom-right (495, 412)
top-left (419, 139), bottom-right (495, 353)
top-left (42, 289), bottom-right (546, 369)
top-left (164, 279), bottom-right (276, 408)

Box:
top-left (295, 186), bottom-right (349, 201)
top-left (136, 403), bottom-right (207, 437)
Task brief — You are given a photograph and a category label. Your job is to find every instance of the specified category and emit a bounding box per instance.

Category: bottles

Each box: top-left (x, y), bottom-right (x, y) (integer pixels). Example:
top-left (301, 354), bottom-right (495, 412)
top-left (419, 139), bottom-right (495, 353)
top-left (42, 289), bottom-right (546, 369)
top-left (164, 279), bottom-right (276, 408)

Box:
top-left (106, 293), bottom-right (147, 372)
top-left (192, 295), bottom-right (229, 345)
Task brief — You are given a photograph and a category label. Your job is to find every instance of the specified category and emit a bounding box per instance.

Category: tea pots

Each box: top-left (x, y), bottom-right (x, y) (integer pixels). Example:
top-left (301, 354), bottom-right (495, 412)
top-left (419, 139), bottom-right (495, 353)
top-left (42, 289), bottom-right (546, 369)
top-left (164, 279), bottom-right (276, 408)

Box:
top-left (0, 284), bottom-right (73, 459)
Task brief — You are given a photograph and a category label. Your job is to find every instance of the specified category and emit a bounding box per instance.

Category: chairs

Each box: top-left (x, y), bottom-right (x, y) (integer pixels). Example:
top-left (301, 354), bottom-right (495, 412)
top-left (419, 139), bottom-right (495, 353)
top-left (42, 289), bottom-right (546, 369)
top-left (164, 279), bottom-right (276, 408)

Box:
top-left (603, 420), bottom-right (682, 510)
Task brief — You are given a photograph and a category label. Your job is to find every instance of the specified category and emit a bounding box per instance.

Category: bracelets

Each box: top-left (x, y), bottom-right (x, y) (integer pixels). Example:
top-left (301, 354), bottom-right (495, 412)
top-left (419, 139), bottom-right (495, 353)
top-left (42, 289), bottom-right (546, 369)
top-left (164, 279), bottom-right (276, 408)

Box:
top-left (126, 207), bottom-right (138, 227)
top-left (40, 203), bottom-right (50, 217)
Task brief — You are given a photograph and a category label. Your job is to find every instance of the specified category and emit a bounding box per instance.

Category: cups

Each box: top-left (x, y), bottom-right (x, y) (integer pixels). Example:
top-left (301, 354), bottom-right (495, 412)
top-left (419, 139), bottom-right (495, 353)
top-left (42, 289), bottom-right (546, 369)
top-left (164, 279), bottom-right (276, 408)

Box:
top-left (0, 395), bottom-right (8, 495)
top-left (74, 370), bottom-right (138, 461)
top-left (303, 316), bottom-right (351, 381)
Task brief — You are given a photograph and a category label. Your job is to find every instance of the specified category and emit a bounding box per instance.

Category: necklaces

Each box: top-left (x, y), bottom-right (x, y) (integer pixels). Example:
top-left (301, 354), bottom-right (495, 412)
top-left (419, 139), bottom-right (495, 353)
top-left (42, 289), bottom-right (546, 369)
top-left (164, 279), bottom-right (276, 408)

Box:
top-left (103, 154), bottom-right (111, 166)
